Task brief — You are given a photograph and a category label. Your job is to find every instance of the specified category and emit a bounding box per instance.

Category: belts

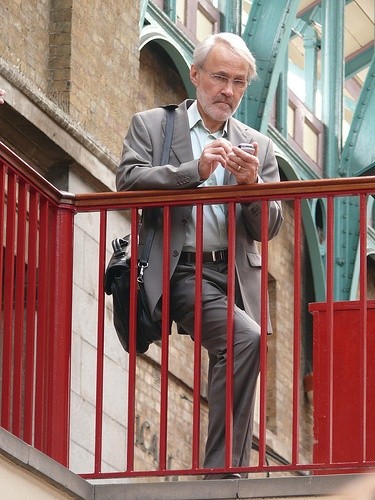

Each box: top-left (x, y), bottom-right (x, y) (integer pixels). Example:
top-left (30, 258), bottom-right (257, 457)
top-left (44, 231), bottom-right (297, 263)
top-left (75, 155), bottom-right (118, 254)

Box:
top-left (179, 250), bottom-right (228, 263)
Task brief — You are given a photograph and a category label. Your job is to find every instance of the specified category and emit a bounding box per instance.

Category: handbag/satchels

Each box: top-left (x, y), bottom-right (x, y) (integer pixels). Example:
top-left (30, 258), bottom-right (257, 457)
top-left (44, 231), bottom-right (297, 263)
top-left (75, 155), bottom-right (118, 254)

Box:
top-left (104, 234), bottom-right (171, 355)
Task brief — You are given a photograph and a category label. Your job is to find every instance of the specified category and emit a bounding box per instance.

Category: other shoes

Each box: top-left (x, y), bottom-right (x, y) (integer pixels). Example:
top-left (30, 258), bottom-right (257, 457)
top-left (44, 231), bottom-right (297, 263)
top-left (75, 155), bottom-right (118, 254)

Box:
top-left (220, 473), bottom-right (242, 480)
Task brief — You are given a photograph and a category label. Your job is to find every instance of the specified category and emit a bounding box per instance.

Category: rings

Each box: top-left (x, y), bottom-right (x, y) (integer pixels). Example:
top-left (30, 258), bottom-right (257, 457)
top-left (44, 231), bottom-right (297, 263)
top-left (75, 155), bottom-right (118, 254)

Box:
top-left (237, 166), bottom-right (241, 172)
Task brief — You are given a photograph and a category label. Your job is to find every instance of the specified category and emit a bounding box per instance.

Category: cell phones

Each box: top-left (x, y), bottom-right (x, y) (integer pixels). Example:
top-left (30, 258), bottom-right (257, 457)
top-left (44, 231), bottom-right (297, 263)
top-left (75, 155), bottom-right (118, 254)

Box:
top-left (238, 143), bottom-right (255, 155)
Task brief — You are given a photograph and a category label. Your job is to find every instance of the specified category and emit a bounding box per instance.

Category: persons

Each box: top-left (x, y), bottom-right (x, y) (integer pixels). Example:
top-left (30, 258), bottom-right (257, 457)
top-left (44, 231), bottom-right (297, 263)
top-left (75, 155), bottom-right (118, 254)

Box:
top-left (115, 32), bottom-right (284, 478)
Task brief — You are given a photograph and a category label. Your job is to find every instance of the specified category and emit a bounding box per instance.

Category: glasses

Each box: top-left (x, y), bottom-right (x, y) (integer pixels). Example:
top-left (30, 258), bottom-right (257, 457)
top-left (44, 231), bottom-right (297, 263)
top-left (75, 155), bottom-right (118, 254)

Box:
top-left (201, 66), bottom-right (251, 89)
